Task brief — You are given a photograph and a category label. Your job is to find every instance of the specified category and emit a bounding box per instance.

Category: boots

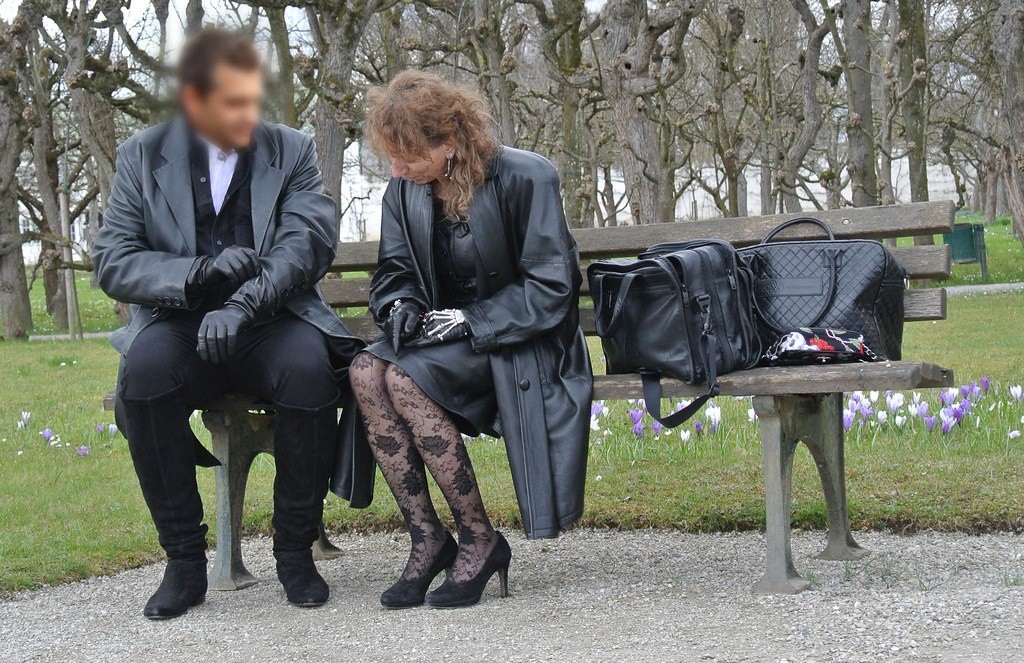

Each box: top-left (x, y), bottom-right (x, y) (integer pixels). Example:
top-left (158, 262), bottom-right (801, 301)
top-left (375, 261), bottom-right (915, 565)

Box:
top-left (119, 384), bottom-right (208, 620)
top-left (272, 388), bottom-right (340, 606)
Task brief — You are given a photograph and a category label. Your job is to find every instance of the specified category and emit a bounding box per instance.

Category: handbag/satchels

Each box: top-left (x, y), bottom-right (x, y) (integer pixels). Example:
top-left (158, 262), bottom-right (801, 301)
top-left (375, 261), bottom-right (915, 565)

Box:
top-left (737, 217), bottom-right (910, 366)
top-left (587, 238), bottom-right (762, 428)
top-left (762, 326), bottom-right (889, 367)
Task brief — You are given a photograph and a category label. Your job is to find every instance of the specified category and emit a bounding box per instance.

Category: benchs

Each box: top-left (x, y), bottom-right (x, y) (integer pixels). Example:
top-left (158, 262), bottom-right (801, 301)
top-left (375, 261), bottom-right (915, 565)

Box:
top-left (102, 199), bottom-right (955, 594)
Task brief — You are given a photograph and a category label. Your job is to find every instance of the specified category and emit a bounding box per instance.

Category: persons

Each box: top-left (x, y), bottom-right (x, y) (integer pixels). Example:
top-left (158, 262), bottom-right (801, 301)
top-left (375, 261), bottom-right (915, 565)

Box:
top-left (349, 68), bottom-right (592, 609)
top-left (89, 25), bottom-right (378, 620)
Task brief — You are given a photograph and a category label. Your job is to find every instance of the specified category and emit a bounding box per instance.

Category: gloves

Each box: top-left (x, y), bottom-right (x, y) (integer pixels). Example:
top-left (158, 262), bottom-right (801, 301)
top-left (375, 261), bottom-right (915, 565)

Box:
top-left (404, 308), bottom-right (472, 348)
top-left (383, 299), bottom-right (423, 356)
top-left (197, 244), bottom-right (261, 291)
top-left (196, 304), bottom-right (250, 365)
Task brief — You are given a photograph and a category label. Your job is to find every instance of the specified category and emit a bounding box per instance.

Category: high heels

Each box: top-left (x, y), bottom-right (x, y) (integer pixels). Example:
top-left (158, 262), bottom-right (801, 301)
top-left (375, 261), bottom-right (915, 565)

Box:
top-left (380, 531), bottom-right (459, 608)
top-left (426, 531), bottom-right (511, 607)
